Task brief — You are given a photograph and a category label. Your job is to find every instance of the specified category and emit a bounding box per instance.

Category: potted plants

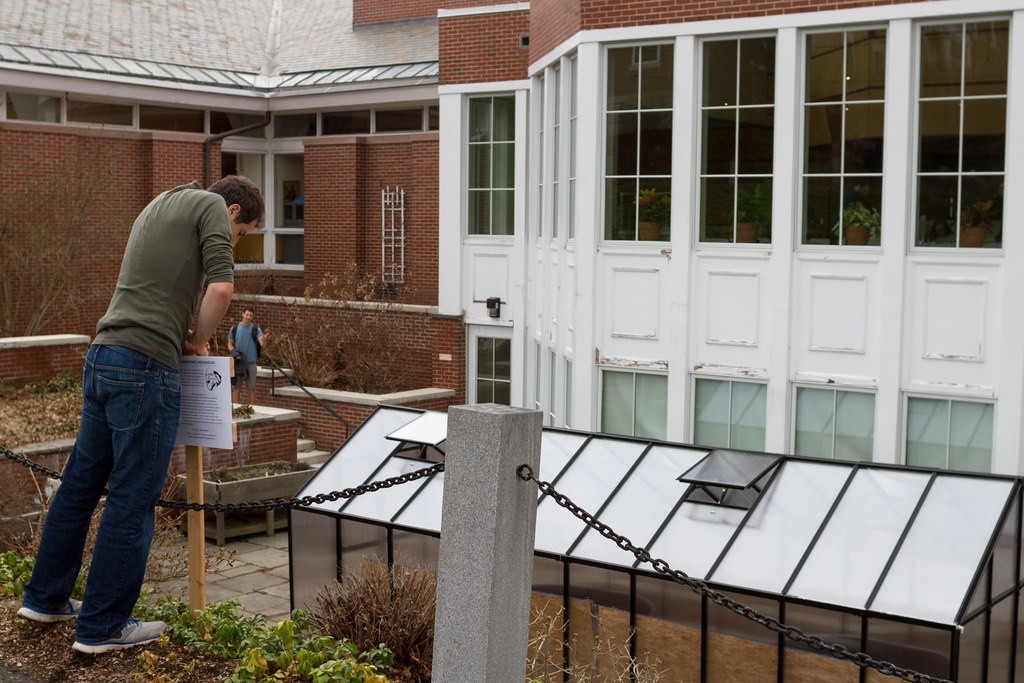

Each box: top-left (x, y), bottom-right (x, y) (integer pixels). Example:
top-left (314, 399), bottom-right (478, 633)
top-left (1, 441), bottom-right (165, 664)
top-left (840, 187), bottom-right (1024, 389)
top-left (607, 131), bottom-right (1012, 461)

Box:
top-left (736, 211), bottom-right (758, 244)
top-left (961, 200), bottom-right (998, 247)
top-left (633, 187), bottom-right (664, 240)
top-left (831, 200), bottom-right (881, 246)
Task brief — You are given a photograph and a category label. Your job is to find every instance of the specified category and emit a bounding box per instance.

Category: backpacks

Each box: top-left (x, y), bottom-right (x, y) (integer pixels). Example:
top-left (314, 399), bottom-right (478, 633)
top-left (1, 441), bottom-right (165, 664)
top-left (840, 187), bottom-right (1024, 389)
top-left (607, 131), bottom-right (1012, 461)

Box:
top-left (232, 324), bottom-right (263, 358)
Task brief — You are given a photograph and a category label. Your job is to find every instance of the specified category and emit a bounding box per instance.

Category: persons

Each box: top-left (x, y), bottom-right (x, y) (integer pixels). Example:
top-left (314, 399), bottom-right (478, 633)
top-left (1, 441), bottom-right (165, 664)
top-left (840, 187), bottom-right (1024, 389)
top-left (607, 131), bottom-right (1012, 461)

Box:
top-left (19, 176), bottom-right (266, 653)
top-left (228, 306), bottom-right (271, 410)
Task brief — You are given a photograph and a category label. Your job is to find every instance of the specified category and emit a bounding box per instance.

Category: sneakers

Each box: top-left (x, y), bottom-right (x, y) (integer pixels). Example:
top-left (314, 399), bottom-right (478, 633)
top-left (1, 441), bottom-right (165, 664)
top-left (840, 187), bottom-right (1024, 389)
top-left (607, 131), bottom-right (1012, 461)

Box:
top-left (73, 620), bottom-right (168, 654)
top-left (17, 596), bottom-right (84, 622)
